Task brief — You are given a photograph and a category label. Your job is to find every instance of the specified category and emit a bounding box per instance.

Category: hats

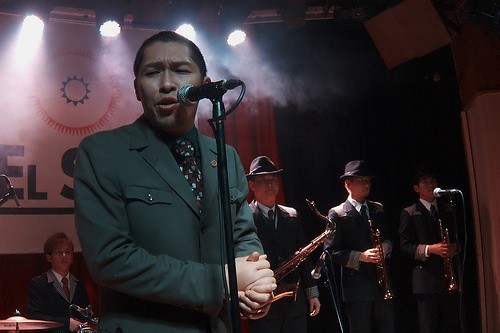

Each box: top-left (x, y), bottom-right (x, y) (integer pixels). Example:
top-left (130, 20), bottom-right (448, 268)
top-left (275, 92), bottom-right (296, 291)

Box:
top-left (245, 156), bottom-right (283, 177)
top-left (340, 161), bottom-right (376, 178)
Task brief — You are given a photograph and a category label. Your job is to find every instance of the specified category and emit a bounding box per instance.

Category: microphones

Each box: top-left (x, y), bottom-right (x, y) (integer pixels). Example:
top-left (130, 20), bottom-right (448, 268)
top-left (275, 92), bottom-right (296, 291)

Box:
top-left (433, 188), bottom-right (461, 198)
top-left (4, 176), bottom-right (20, 207)
top-left (71, 305), bottom-right (98, 325)
top-left (176, 80), bottom-right (243, 107)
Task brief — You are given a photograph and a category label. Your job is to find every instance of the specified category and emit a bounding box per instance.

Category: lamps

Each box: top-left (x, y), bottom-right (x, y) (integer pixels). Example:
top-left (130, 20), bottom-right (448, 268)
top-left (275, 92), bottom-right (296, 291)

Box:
top-left (20, 0), bottom-right (254, 48)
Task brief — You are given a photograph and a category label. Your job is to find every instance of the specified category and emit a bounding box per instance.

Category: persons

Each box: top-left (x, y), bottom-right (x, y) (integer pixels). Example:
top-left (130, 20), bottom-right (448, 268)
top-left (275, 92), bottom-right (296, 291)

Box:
top-left (398, 167), bottom-right (468, 333)
top-left (322, 160), bottom-right (400, 333)
top-left (245, 156), bottom-right (320, 333)
top-left (28, 232), bottom-right (93, 333)
top-left (73, 31), bottom-right (278, 333)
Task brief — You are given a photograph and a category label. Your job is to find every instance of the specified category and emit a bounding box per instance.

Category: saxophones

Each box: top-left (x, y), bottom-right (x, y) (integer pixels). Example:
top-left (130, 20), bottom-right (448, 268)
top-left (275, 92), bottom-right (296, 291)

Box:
top-left (269, 198), bottom-right (337, 301)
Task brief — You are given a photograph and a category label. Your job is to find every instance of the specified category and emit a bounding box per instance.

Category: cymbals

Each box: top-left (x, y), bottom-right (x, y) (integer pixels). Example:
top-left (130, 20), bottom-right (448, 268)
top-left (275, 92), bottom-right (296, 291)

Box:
top-left (0, 314), bottom-right (64, 331)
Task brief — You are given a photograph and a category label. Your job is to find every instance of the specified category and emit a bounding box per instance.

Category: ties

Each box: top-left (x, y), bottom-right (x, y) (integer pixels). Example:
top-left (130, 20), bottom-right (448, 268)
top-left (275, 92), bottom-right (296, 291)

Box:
top-left (170, 138), bottom-right (203, 210)
top-left (60, 277), bottom-right (70, 300)
top-left (268, 210), bottom-right (275, 228)
top-left (430, 204), bottom-right (438, 220)
top-left (360, 205), bottom-right (369, 223)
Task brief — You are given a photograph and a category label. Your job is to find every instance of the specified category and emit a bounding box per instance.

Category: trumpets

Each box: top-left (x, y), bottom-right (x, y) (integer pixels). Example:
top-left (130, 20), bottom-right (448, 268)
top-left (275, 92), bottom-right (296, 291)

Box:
top-left (368, 219), bottom-right (395, 300)
top-left (438, 217), bottom-right (460, 290)
